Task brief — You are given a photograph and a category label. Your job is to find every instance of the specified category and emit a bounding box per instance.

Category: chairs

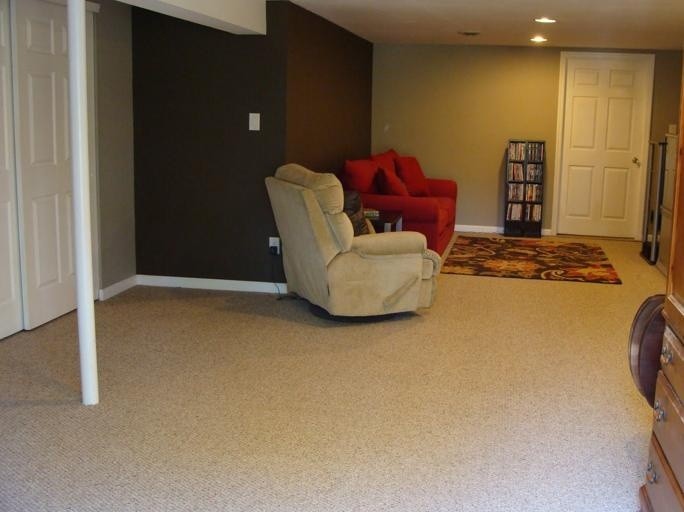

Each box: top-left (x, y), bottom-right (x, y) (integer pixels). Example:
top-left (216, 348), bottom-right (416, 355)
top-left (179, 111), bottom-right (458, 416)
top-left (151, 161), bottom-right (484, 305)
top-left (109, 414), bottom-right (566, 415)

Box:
top-left (262, 161), bottom-right (440, 318)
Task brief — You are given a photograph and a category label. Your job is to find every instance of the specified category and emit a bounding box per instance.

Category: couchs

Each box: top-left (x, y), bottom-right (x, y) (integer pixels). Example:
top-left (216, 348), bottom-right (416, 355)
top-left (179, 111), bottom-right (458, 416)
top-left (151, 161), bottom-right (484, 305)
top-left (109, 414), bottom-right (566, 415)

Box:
top-left (342, 147), bottom-right (458, 257)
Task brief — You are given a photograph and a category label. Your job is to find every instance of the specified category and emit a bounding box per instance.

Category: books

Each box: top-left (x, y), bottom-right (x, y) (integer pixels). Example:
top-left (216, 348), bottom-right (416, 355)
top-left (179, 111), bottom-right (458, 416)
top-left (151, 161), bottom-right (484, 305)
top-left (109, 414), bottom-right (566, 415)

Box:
top-left (363, 217), bottom-right (379, 221)
top-left (506, 143), bottom-right (543, 222)
top-left (362, 208), bottom-right (379, 217)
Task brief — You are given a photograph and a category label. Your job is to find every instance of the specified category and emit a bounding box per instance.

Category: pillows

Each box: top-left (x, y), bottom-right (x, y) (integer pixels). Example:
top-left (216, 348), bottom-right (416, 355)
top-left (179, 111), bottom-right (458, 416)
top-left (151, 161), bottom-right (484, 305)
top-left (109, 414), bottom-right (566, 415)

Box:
top-left (375, 154), bottom-right (432, 198)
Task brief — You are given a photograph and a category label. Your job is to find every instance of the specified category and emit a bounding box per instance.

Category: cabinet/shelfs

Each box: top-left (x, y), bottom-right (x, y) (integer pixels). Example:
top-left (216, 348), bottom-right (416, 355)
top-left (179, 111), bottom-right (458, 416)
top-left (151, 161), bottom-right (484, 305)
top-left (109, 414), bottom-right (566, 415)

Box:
top-left (503, 138), bottom-right (547, 238)
top-left (633, 90), bottom-right (684, 512)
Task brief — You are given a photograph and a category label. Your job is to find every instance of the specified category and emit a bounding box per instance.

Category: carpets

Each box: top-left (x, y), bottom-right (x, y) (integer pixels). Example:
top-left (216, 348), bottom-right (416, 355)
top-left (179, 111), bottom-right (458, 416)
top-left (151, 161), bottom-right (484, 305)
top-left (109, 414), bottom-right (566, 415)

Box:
top-left (442, 235), bottom-right (623, 284)
